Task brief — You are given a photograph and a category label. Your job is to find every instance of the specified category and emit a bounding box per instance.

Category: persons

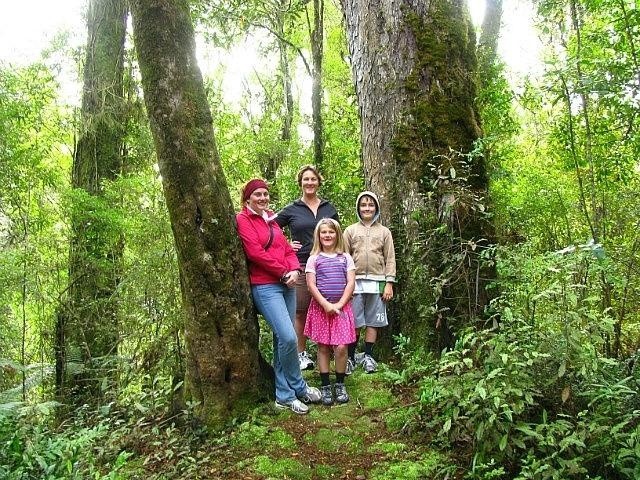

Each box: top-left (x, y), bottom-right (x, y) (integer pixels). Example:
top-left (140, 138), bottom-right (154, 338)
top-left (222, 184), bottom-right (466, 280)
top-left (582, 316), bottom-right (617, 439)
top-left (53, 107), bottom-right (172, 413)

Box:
top-left (235, 179), bottom-right (324, 414)
top-left (273, 164), bottom-right (338, 370)
top-left (301, 218), bottom-right (358, 406)
top-left (344, 189), bottom-right (397, 376)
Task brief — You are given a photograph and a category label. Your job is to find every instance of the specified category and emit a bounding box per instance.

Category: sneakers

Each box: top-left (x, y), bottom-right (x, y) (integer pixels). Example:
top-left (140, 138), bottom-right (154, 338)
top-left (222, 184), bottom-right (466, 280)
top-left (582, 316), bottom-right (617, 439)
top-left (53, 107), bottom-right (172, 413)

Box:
top-left (345, 357), bottom-right (357, 376)
top-left (359, 354), bottom-right (380, 373)
top-left (275, 398), bottom-right (309, 415)
top-left (298, 350), bottom-right (315, 369)
top-left (302, 383), bottom-right (322, 404)
top-left (320, 385), bottom-right (334, 406)
top-left (334, 383), bottom-right (349, 403)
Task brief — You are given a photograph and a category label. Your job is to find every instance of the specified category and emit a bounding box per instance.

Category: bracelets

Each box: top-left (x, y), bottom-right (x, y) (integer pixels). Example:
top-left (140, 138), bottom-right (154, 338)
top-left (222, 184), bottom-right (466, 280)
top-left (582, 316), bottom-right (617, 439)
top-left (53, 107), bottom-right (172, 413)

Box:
top-left (295, 270), bottom-right (302, 277)
